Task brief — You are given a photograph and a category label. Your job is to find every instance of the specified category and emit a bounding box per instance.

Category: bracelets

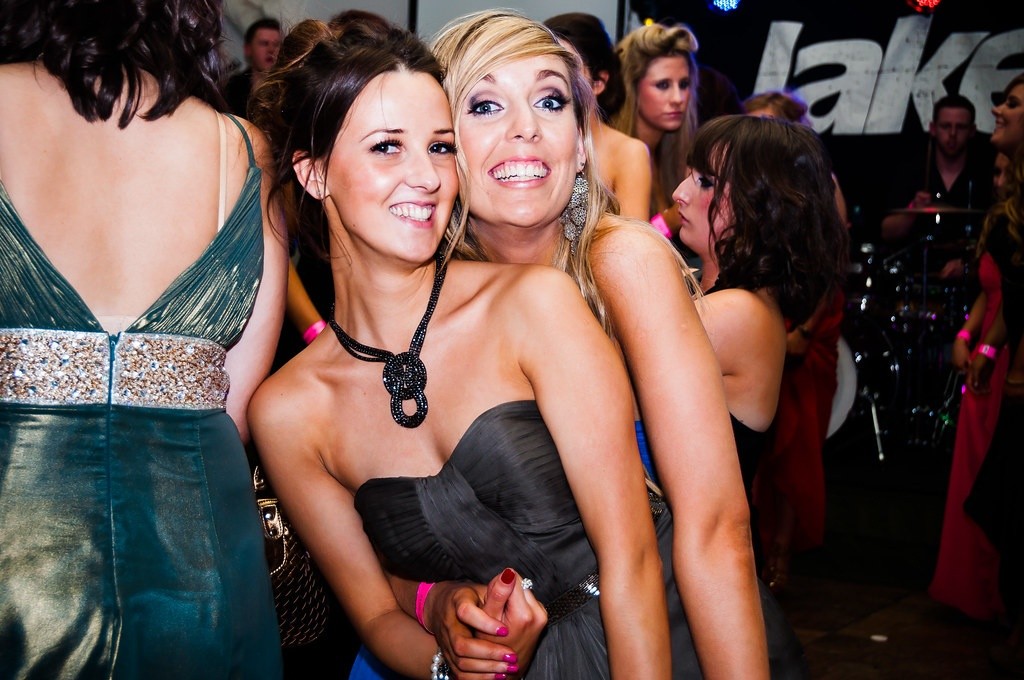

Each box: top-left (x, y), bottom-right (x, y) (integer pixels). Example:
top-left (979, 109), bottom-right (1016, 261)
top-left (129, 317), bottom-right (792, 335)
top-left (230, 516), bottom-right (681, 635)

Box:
top-left (956, 329), bottom-right (970, 341)
top-left (976, 343), bottom-right (996, 358)
top-left (303, 319), bottom-right (327, 344)
top-left (431, 650), bottom-right (449, 680)
top-left (416, 582), bottom-right (436, 634)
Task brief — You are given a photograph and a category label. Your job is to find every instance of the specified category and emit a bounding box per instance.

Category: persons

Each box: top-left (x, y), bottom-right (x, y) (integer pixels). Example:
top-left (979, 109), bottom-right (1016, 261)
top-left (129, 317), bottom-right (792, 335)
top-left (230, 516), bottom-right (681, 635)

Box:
top-left (0, 0), bottom-right (1024, 680)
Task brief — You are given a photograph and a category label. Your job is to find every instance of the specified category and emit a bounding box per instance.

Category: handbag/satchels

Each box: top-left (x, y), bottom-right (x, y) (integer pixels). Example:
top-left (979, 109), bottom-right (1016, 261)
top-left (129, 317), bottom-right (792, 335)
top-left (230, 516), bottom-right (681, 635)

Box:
top-left (251, 464), bottom-right (331, 655)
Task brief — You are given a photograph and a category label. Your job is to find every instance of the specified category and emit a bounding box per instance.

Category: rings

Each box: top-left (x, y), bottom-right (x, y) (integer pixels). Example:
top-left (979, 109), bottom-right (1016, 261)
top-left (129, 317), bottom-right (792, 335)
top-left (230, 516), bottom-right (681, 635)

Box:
top-left (520, 578), bottom-right (533, 590)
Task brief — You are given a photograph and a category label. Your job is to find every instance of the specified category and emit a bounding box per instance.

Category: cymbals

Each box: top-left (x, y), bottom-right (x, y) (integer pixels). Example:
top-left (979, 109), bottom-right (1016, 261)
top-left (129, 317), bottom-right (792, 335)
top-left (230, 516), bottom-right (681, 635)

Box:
top-left (889, 207), bottom-right (985, 214)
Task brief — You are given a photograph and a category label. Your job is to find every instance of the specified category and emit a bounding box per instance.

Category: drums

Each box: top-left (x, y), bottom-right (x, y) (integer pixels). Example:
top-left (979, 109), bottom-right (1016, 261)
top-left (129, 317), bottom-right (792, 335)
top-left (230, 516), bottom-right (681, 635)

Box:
top-left (905, 277), bottom-right (964, 314)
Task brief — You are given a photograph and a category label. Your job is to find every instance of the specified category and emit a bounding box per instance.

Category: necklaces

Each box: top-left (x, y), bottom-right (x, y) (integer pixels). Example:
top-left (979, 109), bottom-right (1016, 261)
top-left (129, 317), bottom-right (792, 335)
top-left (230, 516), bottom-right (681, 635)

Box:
top-left (329, 252), bottom-right (447, 429)
